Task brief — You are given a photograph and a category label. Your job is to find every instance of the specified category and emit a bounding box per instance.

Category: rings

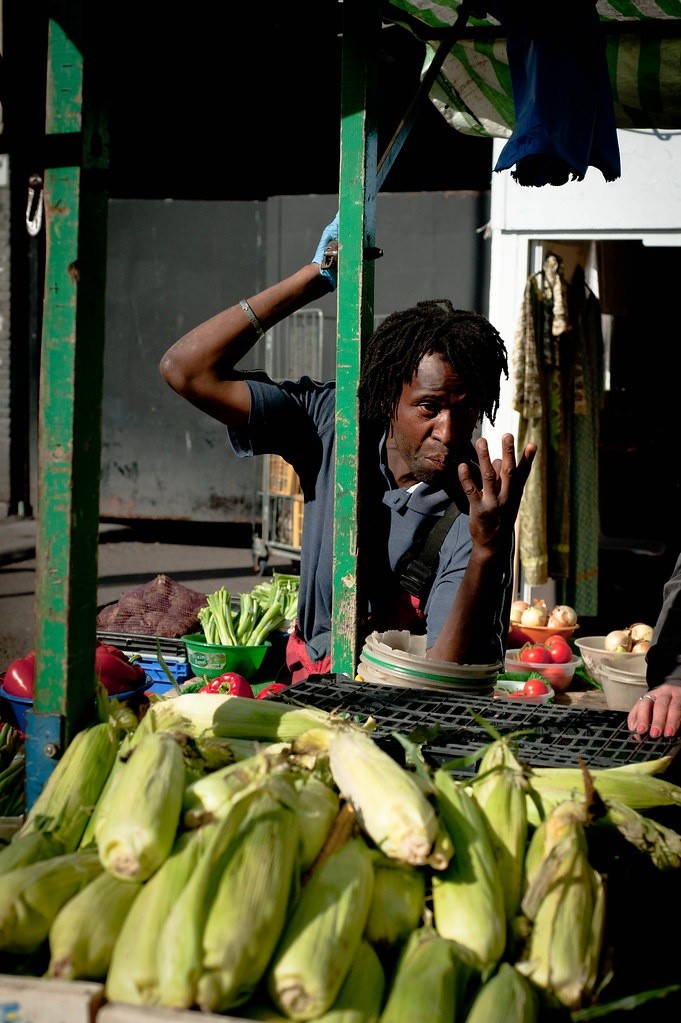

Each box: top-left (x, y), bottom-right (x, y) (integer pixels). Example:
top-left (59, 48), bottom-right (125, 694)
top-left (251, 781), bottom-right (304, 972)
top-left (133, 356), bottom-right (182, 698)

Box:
top-left (639, 694), bottom-right (655, 702)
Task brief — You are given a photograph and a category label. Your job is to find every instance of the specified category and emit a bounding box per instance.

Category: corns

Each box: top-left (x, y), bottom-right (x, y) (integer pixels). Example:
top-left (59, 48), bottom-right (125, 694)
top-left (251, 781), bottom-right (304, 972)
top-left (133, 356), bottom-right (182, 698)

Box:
top-left (0, 695), bottom-right (680, 1023)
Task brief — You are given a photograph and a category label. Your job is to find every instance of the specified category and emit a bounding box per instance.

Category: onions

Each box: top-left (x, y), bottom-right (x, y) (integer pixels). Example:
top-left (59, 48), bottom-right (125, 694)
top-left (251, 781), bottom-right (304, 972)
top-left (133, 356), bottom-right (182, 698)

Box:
top-left (604, 622), bottom-right (655, 654)
top-left (509, 599), bottom-right (577, 628)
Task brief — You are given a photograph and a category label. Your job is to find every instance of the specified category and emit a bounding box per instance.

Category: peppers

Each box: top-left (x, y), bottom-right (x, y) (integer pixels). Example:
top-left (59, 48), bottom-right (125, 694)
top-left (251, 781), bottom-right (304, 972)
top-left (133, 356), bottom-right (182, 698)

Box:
top-left (3, 636), bottom-right (146, 698)
top-left (198, 671), bottom-right (287, 700)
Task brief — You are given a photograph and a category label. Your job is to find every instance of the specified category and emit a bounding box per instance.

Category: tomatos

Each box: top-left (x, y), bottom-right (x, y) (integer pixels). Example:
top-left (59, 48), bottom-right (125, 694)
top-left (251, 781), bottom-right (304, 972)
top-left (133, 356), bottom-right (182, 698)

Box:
top-left (508, 634), bottom-right (573, 698)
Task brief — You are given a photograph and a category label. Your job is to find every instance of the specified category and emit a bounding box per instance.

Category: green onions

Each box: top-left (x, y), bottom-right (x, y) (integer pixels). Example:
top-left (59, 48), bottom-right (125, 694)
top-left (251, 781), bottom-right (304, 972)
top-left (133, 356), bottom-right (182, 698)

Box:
top-left (196, 573), bottom-right (299, 647)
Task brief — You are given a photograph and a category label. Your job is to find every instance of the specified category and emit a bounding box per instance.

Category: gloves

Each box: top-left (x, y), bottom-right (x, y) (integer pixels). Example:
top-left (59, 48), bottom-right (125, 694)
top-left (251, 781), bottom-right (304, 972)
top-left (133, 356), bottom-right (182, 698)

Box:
top-left (310, 209), bottom-right (338, 293)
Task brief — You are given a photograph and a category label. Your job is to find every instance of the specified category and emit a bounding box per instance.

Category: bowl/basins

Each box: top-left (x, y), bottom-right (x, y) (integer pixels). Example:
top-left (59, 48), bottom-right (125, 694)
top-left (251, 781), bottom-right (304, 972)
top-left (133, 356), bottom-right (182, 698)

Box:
top-left (597, 657), bottom-right (651, 712)
top-left (490, 679), bottom-right (555, 705)
top-left (181, 634), bottom-right (273, 682)
top-left (503, 648), bottom-right (582, 692)
top-left (506, 617), bottom-right (580, 646)
top-left (574, 637), bottom-right (647, 684)
top-left (0, 671), bottom-right (154, 733)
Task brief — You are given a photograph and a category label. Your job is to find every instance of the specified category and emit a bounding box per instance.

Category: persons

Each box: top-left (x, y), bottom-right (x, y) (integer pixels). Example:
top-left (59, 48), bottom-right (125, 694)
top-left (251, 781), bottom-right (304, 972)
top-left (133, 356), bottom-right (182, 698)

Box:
top-left (626, 554), bottom-right (681, 739)
top-left (161, 213), bottom-right (539, 684)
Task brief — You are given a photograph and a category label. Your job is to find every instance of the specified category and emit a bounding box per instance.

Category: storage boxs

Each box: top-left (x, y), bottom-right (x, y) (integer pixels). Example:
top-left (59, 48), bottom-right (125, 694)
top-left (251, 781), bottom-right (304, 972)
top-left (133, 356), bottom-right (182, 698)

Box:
top-left (98, 597), bottom-right (193, 694)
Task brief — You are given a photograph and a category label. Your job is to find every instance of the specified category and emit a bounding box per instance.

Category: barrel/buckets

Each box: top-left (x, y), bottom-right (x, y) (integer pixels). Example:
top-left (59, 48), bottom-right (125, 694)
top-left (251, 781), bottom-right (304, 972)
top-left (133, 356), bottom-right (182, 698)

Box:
top-left (355, 628), bottom-right (503, 698)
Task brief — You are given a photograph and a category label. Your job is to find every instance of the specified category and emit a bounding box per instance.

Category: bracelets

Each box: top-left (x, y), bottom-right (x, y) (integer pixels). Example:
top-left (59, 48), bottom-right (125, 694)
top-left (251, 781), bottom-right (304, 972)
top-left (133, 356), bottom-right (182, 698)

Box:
top-left (240, 298), bottom-right (265, 340)
